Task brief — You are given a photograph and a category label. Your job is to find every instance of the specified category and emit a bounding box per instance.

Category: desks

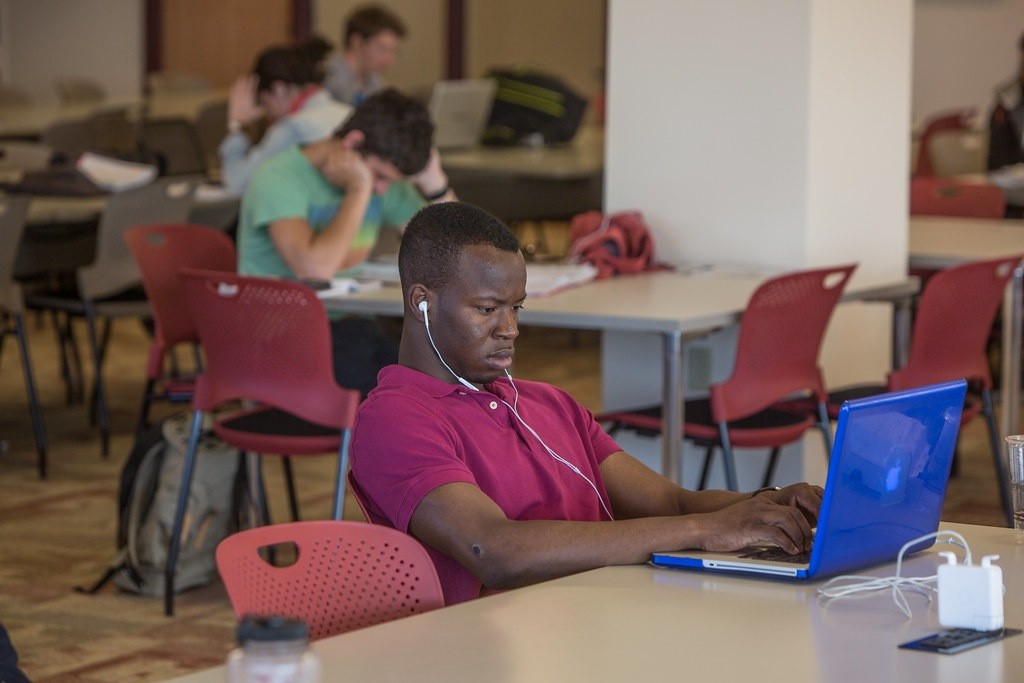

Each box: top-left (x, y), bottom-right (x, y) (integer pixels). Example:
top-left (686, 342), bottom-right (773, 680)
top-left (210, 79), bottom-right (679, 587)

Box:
top-left (439, 129), bottom-right (602, 227)
top-left (246, 256), bottom-right (921, 569)
top-left (2, 187), bottom-right (244, 291)
top-left (908, 161), bottom-right (1024, 520)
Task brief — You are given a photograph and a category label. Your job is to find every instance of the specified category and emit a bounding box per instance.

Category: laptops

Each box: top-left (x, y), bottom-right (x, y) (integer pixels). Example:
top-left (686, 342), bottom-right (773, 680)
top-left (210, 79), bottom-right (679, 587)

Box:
top-left (427, 76), bottom-right (497, 151)
top-left (651, 378), bottom-right (969, 579)
top-left (137, 116), bottom-right (223, 184)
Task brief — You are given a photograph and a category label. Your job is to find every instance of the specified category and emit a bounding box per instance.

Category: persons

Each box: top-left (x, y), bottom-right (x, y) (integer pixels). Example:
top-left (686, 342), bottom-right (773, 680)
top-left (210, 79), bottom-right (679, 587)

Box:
top-left (239, 89), bottom-right (458, 401)
top-left (350, 202), bottom-right (826, 607)
top-left (987, 34), bottom-right (1024, 172)
top-left (218, 38), bottom-right (355, 198)
top-left (327, 8), bottom-right (409, 105)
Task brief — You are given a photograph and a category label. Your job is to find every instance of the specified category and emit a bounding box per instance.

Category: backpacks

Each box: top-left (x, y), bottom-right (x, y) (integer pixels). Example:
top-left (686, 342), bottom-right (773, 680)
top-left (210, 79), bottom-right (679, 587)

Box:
top-left (68, 407), bottom-right (264, 595)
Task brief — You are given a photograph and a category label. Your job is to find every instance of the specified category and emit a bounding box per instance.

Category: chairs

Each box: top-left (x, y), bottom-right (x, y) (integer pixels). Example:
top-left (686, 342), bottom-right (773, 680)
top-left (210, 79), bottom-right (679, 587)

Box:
top-left (137, 521), bottom-right (1024, 683)
top-left (2, 179), bottom-right (195, 477)
top-left (163, 269), bottom-right (364, 616)
top-left (759, 255), bottom-right (1023, 528)
top-left (37, 95), bottom-right (236, 185)
top-left (117, 224), bottom-right (304, 569)
top-left (216, 471), bottom-right (445, 650)
top-left (911, 178), bottom-right (1006, 220)
top-left (588, 263), bottom-right (833, 492)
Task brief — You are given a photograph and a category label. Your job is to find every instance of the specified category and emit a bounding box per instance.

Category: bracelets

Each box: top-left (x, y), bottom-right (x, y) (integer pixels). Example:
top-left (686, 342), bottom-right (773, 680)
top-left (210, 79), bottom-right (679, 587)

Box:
top-left (426, 188), bottom-right (448, 201)
top-left (749, 487), bottom-right (781, 498)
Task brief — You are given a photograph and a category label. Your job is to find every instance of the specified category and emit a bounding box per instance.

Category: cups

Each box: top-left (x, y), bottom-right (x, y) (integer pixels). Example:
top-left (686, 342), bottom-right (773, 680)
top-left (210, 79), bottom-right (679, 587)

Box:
top-left (1003, 434), bottom-right (1024, 545)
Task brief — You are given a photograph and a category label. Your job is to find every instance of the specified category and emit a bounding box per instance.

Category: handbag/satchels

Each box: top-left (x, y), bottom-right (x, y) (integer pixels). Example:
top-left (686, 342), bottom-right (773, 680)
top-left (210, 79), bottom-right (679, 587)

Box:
top-left (483, 62), bottom-right (589, 149)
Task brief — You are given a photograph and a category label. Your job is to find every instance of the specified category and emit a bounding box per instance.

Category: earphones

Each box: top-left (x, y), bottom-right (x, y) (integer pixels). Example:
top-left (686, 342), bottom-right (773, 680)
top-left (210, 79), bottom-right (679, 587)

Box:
top-left (419, 301), bottom-right (429, 325)
